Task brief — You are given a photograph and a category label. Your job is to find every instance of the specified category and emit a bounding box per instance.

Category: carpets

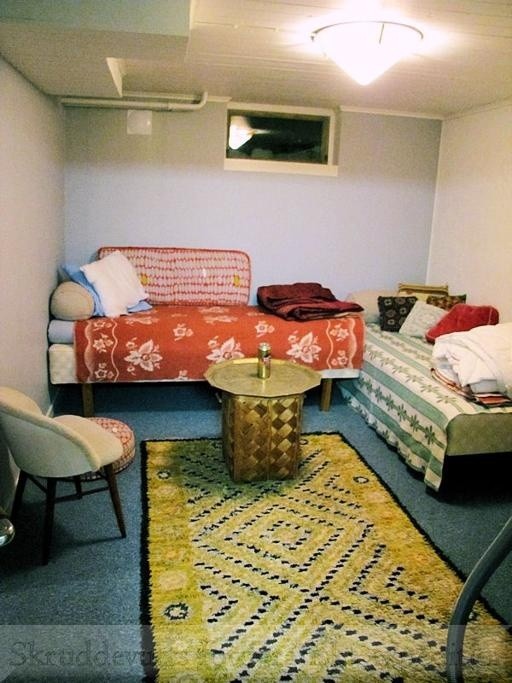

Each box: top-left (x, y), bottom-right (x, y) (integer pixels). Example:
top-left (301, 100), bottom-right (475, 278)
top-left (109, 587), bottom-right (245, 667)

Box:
top-left (140, 429), bottom-right (512, 683)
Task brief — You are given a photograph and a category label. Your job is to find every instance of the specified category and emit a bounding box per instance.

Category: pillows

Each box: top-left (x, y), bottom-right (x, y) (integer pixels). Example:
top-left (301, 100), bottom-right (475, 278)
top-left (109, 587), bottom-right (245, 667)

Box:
top-left (347, 282), bottom-right (500, 347)
top-left (49, 250), bottom-right (153, 321)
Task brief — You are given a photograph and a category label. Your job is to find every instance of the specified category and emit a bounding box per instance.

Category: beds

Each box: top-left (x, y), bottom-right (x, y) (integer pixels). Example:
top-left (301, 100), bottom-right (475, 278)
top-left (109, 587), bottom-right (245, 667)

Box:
top-left (47, 303), bottom-right (362, 419)
top-left (337, 322), bottom-right (512, 473)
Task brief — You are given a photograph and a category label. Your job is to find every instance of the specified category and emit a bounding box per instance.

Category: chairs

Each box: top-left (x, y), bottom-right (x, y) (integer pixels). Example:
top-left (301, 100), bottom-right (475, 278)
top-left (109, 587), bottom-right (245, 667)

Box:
top-left (1, 384), bottom-right (127, 566)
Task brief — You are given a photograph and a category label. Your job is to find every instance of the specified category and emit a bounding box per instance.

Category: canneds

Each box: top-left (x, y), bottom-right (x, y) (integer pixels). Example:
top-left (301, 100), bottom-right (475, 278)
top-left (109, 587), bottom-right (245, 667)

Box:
top-left (257, 342), bottom-right (271, 379)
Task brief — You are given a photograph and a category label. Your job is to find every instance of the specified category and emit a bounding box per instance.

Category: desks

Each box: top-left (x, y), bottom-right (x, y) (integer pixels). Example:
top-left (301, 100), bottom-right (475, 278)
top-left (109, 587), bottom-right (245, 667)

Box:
top-left (204, 357), bottom-right (323, 483)
